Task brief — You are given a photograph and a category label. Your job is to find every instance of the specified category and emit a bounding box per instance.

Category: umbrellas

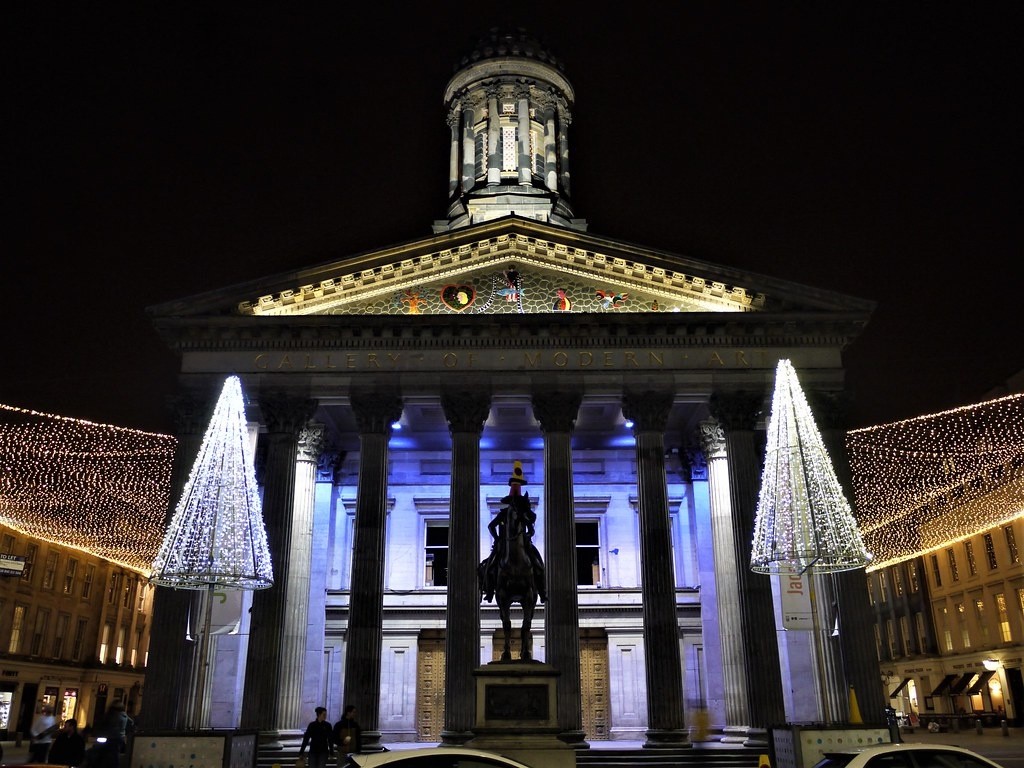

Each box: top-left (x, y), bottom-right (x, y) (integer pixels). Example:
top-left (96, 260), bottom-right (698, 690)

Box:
top-left (148, 373), bottom-right (273, 732)
top-left (748, 358), bottom-right (872, 722)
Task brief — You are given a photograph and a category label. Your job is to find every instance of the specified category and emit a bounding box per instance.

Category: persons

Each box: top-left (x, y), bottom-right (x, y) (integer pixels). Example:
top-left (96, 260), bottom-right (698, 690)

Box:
top-left (101, 699), bottom-right (134, 768)
top-left (27, 705), bottom-right (56, 763)
top-left (48, 719), bottom-right (85, 768)
top-left (479, 494), bottom-right (547, 603)
top-left (333, 705), bottom-right (362, 768)
top-left (298, 707), bottom-right (334, 768)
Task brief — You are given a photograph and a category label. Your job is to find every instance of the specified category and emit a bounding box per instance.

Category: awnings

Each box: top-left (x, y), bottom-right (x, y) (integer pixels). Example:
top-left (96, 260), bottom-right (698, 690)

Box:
top-left (931, 674), bottom-right (957, 697)
top-left (948, 673), bottom-right (976, 696)
top-left (890, 677), bottom-right (911, 698)
top-left (967, 671), bottom-right (996, 695)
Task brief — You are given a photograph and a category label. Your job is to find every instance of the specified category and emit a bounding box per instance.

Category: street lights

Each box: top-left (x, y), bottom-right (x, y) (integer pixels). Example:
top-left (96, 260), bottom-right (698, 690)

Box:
top-left (881, 671), bottom-right (893, 686)
top-left (54, 676), bottom-right (66, 727)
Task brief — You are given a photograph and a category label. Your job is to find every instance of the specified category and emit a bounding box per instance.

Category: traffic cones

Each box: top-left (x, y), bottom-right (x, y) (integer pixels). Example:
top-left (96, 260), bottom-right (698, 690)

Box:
top-left (846, 684), bottom-right (865, 725)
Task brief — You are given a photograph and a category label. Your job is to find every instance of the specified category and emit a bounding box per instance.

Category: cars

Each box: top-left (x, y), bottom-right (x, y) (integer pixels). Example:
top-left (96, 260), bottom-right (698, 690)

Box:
top-left (341, 747), bottom-right (535, 768)
top-left (812, 744), bottom-right (1006, 768)
top-left (94, 717), bottom-right (136, 752)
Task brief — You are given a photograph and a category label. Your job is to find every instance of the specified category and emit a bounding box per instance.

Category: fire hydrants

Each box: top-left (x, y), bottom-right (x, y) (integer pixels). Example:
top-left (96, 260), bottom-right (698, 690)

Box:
top-left (1000, 720), bottom-right (1009, 736)
top-left (975, 719), bottom-right (984, 735)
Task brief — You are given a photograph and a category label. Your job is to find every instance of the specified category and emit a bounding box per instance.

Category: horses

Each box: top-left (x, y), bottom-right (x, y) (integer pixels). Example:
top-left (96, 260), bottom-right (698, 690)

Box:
top-left (494, 491), bottom-right (541, 661)
